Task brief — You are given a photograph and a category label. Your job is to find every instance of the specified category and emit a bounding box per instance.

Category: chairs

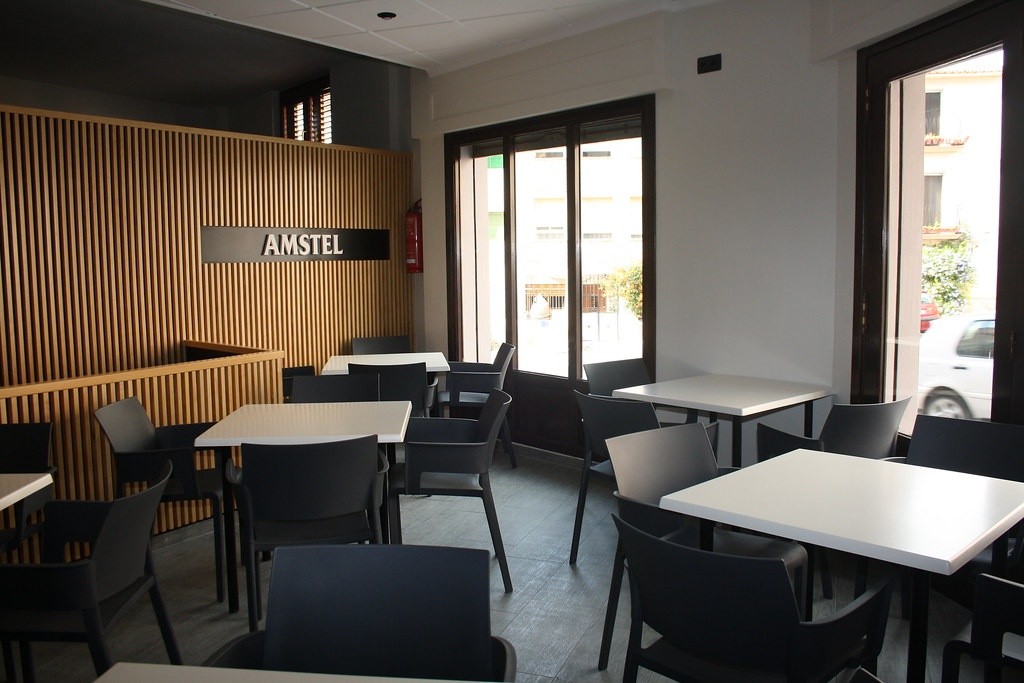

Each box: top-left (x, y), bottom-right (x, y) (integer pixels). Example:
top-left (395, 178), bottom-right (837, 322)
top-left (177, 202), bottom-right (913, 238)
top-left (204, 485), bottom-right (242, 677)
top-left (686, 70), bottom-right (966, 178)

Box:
top-left (0, 334), bottom-right (1024, 683)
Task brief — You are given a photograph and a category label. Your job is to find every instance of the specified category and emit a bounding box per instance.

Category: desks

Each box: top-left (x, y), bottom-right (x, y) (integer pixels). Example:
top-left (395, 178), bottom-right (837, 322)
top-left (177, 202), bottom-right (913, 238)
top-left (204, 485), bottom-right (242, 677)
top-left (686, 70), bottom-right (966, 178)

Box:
top-left (194, 399), bottom-right (414, 616)
top-left (611, 372), bottom-right (838, 467)
top-left (658, 448), bottom-right (1024, 683)
top-left (319, 351), bottom-right (451, 417)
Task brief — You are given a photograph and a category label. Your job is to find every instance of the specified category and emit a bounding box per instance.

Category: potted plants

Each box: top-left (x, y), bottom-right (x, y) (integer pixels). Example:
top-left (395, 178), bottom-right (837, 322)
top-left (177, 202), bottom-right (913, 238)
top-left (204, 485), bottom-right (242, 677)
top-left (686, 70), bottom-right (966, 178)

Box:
top-left (924, 132), bottom-right (932, 145)
top-left (932, 134), bottom-right (940, 145)
top-left (922, 216), bottom-right (959, 234)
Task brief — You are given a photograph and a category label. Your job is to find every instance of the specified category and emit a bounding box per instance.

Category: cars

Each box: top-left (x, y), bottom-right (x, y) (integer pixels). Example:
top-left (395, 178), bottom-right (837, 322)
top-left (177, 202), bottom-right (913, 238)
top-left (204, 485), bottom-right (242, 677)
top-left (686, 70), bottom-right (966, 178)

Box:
top-left (918, 292), bottom-right (996, 420)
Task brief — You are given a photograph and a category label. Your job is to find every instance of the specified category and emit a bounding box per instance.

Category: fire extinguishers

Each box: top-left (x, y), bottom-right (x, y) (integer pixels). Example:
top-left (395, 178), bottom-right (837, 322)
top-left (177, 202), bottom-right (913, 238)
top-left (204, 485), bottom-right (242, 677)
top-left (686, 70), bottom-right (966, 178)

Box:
top-left (405, 198), bottom-right (423, 273)
